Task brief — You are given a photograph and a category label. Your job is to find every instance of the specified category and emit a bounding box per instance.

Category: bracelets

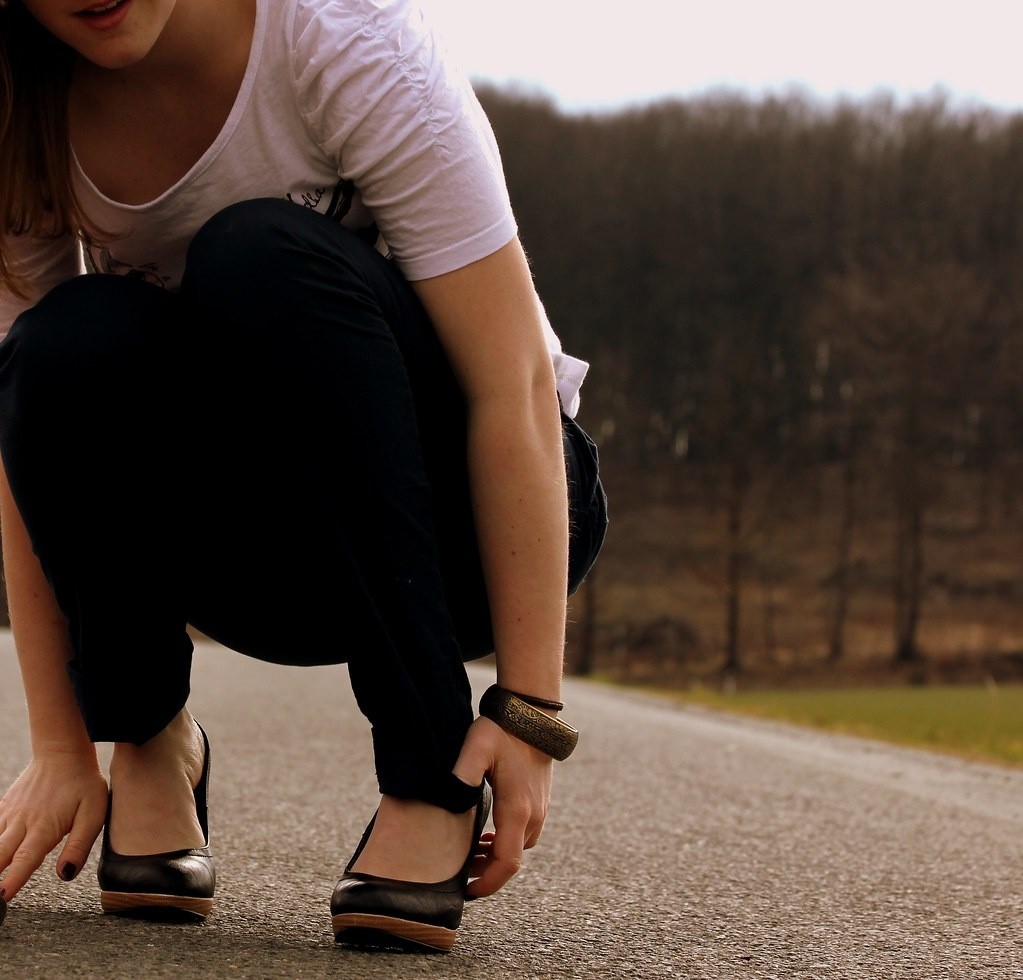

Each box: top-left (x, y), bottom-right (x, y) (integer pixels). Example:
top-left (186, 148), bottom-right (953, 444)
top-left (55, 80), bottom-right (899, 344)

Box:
top-left (478, 685), bottom-right (579, 761)
top-left (507, 689), bottom-right (564, 711)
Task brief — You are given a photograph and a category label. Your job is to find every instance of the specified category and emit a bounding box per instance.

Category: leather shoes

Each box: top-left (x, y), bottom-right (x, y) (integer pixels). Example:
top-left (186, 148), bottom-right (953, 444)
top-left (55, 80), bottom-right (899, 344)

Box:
top-left (96, 717), bottom-right (216, 923)
top-left (329, 772), bottom-right (498, 952)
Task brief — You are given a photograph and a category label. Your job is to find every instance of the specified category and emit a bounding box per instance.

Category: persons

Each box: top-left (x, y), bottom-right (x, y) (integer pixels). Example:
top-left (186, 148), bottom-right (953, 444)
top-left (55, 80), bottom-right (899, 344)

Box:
top-left (0, 0), bottom-right (612, 951)
